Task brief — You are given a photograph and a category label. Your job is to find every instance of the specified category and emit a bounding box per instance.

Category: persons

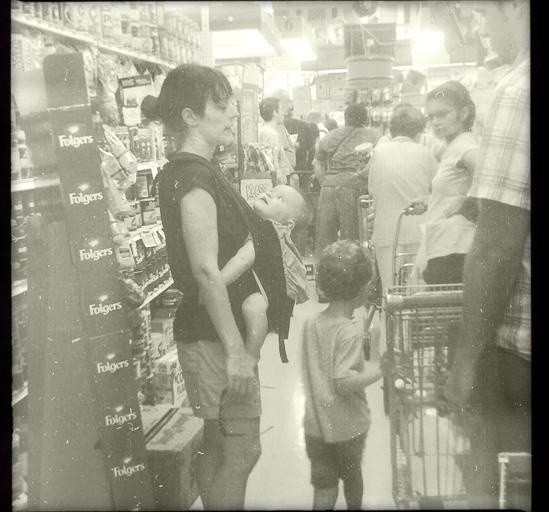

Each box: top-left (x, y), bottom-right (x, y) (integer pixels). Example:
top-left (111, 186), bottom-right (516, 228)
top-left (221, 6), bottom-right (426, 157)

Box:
top-left (138, 64), bottom-right (266, 509)
top-left (401, 79), bottom-right (489, 421)
top-left (256, 89), bottom-right (450, 391)
top-left (196, 181), bottom-right (310, 371)
top-left (299, 241), bottom-right (396, 510)
top-left (440, 1), bottom-right (532, 509)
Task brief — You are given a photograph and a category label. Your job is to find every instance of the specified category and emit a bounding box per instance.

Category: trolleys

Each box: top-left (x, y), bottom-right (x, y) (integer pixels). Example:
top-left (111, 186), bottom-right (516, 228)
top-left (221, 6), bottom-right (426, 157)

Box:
top-left (355, 194), bottom-right (381, 360)
top-left (285, 170), bottom-right (321, 276)
top-left (377, 204), bottom-right (474, 510)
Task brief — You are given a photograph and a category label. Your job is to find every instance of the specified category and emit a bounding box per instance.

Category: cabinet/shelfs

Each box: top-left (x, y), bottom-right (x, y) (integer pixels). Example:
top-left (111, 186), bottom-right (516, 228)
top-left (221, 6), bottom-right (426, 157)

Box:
top-left (12, 16), bottom-right (276, 511)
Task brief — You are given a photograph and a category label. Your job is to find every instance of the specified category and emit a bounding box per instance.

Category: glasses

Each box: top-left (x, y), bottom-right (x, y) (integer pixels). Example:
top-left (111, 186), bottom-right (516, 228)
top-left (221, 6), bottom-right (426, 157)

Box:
top-left (219, 385), bottom-right (274, 438)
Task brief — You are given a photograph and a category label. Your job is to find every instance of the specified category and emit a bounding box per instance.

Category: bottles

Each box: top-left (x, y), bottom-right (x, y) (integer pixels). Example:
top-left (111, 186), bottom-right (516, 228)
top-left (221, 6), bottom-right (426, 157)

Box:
top-left (13, 2), bottom-right (201, 66)
top-left (12, 195), bottom-right (37, 279)
top-left (394, 378), bottom-right (426, 401)
top-left (11, 129), bottom-right (30, 180)
